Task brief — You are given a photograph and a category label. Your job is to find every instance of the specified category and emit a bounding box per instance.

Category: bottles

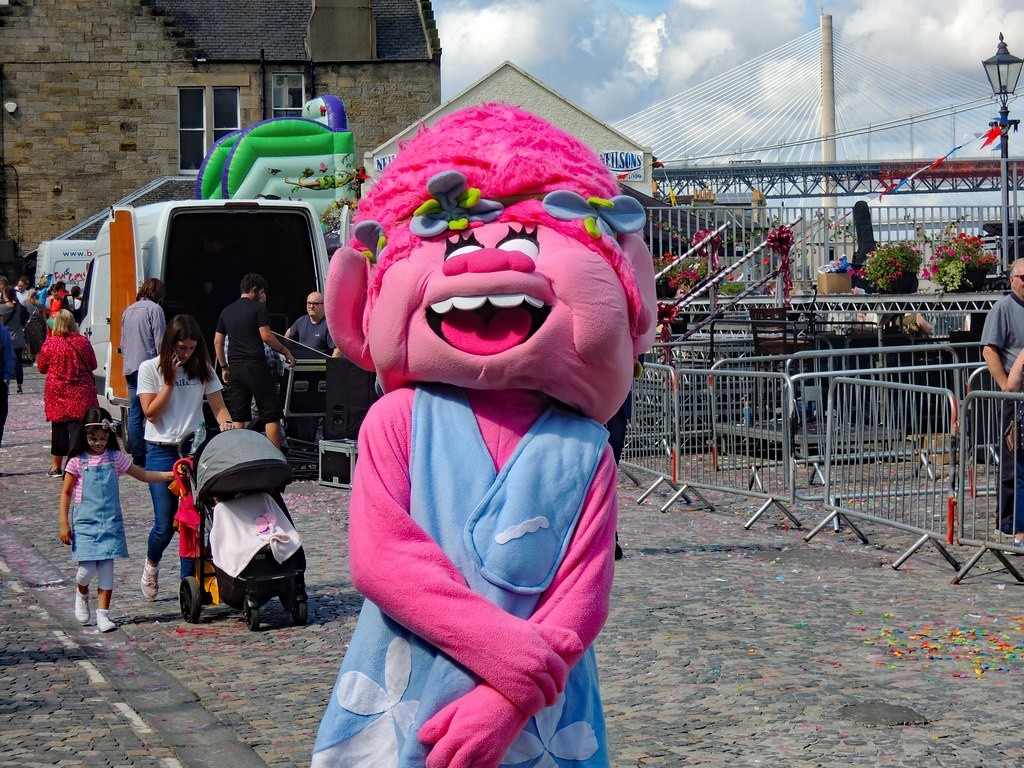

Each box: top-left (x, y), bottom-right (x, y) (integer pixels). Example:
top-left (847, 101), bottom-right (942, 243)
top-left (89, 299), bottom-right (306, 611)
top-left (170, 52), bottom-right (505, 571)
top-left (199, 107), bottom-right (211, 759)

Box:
top-left (190, 421), bottom-right (206, 453)
top-left (744, 401), bottom-right (751, 427)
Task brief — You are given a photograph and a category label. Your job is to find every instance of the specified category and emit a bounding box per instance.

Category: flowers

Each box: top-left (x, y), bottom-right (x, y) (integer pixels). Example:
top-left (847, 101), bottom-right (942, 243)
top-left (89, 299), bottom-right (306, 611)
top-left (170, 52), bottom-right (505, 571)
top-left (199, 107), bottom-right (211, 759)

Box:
top-left (848, 238), bottom-right (927, 293)
top-left (919, 230), bottom-right (1000, 293)
top-left (652, 251), bottom-right (722, 294)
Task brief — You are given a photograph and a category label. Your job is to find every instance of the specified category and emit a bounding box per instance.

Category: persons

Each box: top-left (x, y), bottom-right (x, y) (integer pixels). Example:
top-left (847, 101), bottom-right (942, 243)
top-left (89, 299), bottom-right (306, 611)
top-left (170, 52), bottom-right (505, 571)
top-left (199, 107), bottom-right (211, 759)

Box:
top-left (38, 309), bottom-right (100, 477)
top-left (119, 278), bottom-right (167, 469)
top-left (59, 407), bottom-right (184, 632)
top-left (0, 322), bottom-right (17, 445)
top-left (896, 312), bottom-right (931, 338)
top-left (1006, 348), bottom-right (1024, 556)
top-left (0, 276), bottom-right (83, 367)
top-left (214, 272), bottom-right (296, 451)
top-left (981, 258), bottom-right (1024, 538)
top-left (285, 291), bottom-right (344, 358)
top-left (224, 292), bottom-right (292, 451)
top-left (135, 314), bottom-right (235, 600)
top-left (600, 353), bottom-right (645, 560)
top-left (0, 286), bottom-right (24, 393)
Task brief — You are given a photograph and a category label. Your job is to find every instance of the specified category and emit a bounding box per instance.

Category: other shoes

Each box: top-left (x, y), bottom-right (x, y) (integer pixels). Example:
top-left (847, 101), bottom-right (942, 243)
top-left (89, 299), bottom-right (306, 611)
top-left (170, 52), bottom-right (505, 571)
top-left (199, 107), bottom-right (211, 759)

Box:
top-left (1003, 539), bottom-right (1024, 555)
top-left (141, 558), bottom-right (158, 600)
top-left (96, 611), bottom-right (117, 633)
top-left (46, 469), bottom-right (63, 478)
top-left (75, 586), bottom-right (90, 624)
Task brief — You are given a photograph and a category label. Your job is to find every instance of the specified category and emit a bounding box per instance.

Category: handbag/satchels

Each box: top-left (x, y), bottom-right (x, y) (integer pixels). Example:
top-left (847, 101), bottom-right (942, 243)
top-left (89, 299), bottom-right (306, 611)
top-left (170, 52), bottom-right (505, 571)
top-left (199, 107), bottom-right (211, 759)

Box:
top-left (1004, 420), bottom-right (1018, 452)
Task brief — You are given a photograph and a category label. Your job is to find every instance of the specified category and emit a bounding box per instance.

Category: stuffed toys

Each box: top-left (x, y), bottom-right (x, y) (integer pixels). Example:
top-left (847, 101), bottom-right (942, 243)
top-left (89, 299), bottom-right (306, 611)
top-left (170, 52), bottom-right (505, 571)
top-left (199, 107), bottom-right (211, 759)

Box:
top-left (312, 104), bottom-right (661, 768)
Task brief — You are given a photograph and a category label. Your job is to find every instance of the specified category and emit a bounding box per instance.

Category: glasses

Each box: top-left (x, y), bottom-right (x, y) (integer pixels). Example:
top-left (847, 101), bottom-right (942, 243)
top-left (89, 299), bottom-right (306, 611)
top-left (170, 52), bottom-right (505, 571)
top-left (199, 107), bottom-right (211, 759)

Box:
top-left (1010, 275), bottom-right (1024, 283)
top-left (306, 302), bottom-right (323, 307)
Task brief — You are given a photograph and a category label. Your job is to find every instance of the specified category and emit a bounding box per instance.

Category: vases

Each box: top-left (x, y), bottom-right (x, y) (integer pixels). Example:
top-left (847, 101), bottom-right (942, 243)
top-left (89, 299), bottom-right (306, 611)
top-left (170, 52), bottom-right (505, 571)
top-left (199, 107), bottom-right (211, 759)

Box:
top-left (656, 274), bottom-right (678, 300)
top-left (866, 269), bottom-right (920, 296)
top-left (683, 276), bottom-right (711, 299)
top-left (939, 260), bottom-right (994, 294)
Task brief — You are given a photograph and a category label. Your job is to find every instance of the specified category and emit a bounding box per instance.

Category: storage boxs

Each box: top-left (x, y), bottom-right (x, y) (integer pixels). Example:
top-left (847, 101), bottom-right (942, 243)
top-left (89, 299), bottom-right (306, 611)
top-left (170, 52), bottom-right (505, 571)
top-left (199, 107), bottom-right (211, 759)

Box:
top-left (268, 343), bottom-right (334, 419)
top-left (816, 272), bottom-right (853, 296)
top-left (318, 437), bottom-right (360, 491)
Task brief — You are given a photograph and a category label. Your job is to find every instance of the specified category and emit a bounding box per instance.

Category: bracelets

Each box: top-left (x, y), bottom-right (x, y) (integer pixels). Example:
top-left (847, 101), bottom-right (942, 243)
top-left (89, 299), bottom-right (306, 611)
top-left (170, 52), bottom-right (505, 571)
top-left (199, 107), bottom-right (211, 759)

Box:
top-left (277, 344), bottom-right (284, 354)
top-left (221, 364), bottom-right (228, 368)
top-left (226, 420), bottom-right (233, 423)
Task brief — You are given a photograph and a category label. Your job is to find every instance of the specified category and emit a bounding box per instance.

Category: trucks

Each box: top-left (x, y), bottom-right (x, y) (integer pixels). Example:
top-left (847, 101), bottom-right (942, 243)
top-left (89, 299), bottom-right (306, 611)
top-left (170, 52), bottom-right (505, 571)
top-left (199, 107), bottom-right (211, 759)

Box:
top-left (35, 240), bottom-right (97, 309)
top-left (78, 198), bottom-right (333, 454)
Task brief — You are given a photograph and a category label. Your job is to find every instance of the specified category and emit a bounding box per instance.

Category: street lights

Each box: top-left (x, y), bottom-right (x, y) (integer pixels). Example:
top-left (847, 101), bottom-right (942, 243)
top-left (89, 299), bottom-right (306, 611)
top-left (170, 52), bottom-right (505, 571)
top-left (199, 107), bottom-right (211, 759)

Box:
top-left (980, 32), bottom-right (1024, 275)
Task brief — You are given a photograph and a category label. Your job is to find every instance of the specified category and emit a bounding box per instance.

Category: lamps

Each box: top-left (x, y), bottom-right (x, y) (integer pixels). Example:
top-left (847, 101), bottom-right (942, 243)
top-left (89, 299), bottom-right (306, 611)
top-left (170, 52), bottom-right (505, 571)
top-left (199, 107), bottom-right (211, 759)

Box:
top-left (5, 102), bottom-right (18, 115)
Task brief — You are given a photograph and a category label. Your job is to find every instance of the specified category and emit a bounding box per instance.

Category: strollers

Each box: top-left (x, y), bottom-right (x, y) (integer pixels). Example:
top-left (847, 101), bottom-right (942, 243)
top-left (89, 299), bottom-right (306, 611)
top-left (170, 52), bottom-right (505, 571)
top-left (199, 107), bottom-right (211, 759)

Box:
top-left (170, 424), bottom-right (310, 632)
